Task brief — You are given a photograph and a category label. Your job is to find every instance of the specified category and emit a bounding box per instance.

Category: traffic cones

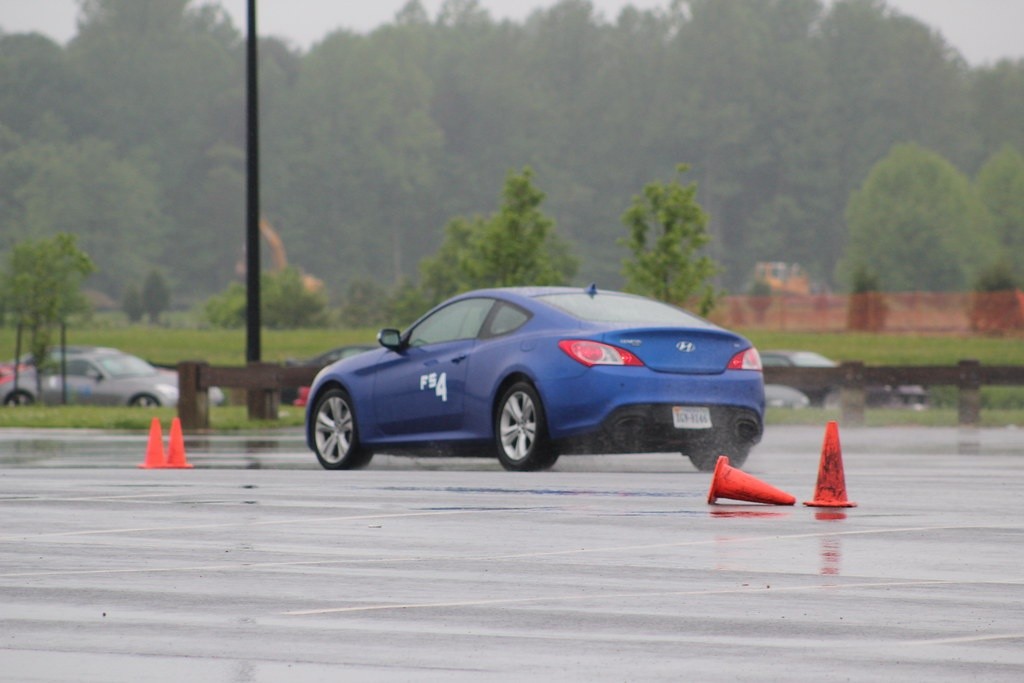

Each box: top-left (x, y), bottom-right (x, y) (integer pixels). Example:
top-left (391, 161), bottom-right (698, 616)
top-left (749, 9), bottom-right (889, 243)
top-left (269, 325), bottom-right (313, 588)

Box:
top-left (138, 416), bottom-right (169, 468)
top-left (707, 455), bottom-right (797, 506)
top-left (164, 417), bottom-right (195, 469)
top-left (802, 420), bottom-right (857, 506)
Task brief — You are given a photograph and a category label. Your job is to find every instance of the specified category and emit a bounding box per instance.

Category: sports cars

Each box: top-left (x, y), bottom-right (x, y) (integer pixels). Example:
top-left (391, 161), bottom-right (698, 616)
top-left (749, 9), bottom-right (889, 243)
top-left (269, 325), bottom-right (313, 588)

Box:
top-left (304, 283), bottom-right (767, 473)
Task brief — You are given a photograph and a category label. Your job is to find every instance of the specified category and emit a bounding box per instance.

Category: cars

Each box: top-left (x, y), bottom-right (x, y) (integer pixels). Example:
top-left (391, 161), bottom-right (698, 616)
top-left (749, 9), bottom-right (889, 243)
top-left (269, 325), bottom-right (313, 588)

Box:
top-left (1, 343), bottom-right (227, 420)
top-left (756, 349), bottom-right (846, 409)
top-left (294, 343), bottom-right (380, 388)
